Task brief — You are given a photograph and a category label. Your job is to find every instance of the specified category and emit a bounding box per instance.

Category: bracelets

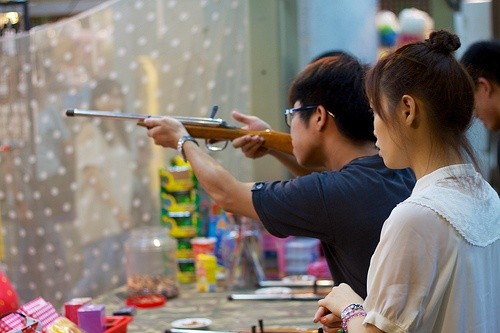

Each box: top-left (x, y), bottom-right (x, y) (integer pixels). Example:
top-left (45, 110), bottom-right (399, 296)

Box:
top-left (342, 311), bottom-right (367, 332)
top-left (177, 135), bottom-right (200, 163)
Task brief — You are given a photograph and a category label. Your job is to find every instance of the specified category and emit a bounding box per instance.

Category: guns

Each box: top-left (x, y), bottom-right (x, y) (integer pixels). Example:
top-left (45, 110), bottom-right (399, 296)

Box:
top-left (66, 104), bottom-right (310, 177)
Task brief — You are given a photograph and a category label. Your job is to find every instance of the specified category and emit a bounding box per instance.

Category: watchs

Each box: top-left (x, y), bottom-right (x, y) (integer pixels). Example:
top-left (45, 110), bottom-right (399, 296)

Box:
top-left (341, 304), bottom-right (364, 319)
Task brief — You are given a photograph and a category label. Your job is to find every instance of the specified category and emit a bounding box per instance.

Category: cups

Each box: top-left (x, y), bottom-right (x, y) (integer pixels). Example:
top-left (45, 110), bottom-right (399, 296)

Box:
top-left (220, 224), bottom-right (267, 293)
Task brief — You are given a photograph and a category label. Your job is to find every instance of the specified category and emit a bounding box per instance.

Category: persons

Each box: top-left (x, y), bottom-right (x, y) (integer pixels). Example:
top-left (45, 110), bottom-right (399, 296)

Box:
top-left (313, 31), bottom-right (500, 333)
top-left (143, 55), bottom-right (417, 301)
top-left (460, 40), bottom-right (500, 131)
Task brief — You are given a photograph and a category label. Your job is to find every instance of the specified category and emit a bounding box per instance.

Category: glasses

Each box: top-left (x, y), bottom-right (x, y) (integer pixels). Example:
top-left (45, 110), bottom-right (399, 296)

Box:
top-left (284, 106), bottom-right (335, 128)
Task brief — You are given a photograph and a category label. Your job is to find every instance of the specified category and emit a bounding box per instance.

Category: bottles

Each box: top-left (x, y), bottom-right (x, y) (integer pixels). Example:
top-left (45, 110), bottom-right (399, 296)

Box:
top-left (124, 225), bottom-right (180, 298)
